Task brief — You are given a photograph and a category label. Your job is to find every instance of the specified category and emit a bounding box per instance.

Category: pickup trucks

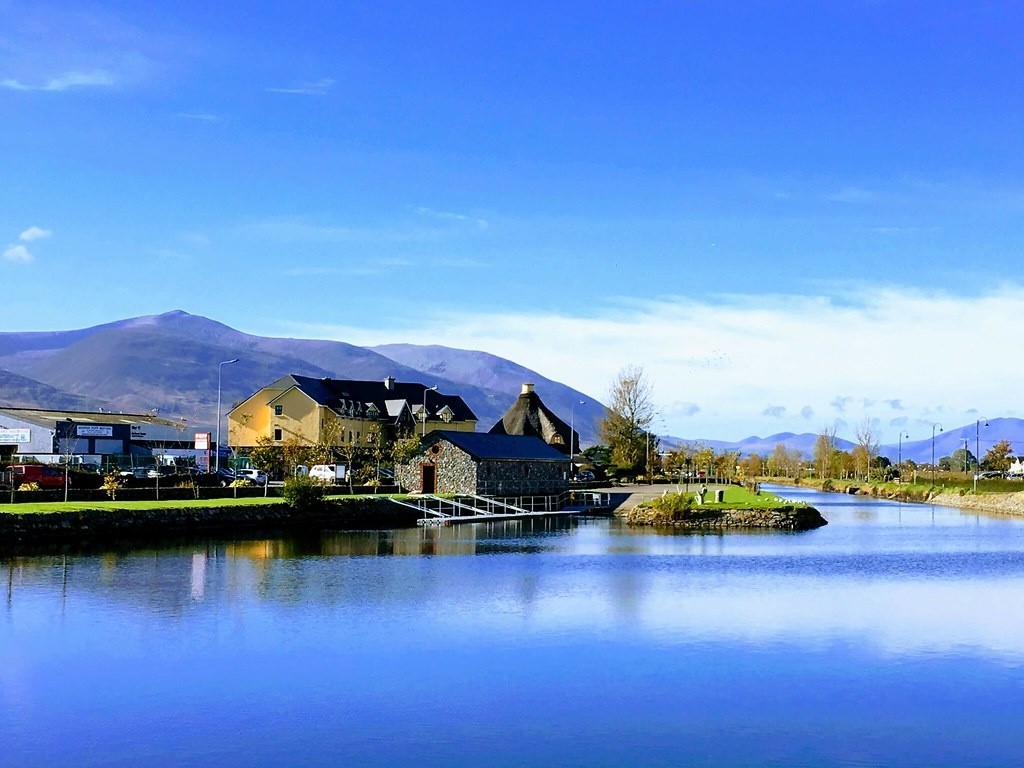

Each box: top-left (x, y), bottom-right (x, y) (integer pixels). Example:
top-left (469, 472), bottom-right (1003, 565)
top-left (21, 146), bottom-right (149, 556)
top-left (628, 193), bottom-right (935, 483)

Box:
top-left (7, 464), bottom-right (72, 490)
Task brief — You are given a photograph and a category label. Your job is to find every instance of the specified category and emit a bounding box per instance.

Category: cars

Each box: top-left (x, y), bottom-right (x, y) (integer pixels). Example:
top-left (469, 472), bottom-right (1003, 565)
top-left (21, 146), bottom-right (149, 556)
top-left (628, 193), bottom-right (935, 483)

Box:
top-left (578, 471), bottom-right (595, 481)
top-left (378, 468), bottom-right (394, 479)
top-left (978, 471), bottom-right (1024, 480)
top-left (77, 463), bottom-right (269, 487)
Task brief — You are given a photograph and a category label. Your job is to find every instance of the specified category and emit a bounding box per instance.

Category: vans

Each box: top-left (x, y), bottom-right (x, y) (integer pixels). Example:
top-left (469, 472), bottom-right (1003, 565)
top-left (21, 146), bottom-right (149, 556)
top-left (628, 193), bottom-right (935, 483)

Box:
top-left (309, 464), bottom-right (346, 484)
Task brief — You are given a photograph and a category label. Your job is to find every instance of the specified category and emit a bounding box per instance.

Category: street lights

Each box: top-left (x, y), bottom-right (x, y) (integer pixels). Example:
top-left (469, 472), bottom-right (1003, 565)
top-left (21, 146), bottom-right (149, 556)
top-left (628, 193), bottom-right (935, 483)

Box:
top-left (932, 422), bottom-right (944, 485)
top-left (215, 358), bottom-right (241, 473)
top-left (422, 384), bottom-right (439, 436)
top-left (976, 417), bottom-right (990, 483)
top-left (646, 419), bottom-right (666, 465)
top-left (898, 428), bottom-right (910, 485)
top-left (570, 400), bottom-right (586, 478)
